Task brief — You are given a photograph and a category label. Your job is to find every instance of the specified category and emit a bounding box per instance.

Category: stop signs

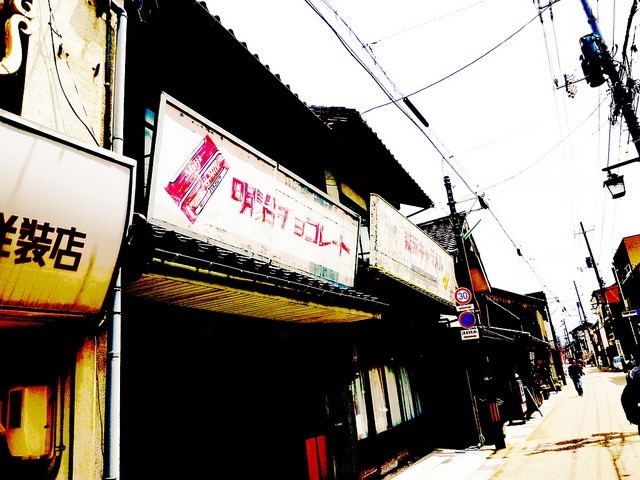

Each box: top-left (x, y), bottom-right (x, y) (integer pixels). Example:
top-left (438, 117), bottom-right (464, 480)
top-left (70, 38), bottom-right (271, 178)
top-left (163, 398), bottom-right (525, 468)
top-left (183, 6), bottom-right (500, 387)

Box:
top-left (459, 311), bottom-right (476, 328)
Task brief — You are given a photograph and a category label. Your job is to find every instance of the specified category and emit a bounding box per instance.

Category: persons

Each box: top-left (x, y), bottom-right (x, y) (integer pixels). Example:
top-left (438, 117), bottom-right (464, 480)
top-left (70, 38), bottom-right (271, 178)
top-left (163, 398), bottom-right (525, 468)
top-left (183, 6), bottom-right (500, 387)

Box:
top-left (568, 359), bottom-right (584, 397)
top-left (621, 344), bottom-right (640, 437)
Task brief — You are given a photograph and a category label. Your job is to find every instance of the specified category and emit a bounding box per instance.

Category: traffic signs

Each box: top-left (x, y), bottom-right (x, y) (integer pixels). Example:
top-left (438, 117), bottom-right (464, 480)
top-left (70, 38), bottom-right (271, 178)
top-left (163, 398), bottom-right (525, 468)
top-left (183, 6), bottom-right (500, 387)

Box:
top-left (461, 329), bottom-right (480, 341)
top-left (455, 288), bottom-right (472, 305)
